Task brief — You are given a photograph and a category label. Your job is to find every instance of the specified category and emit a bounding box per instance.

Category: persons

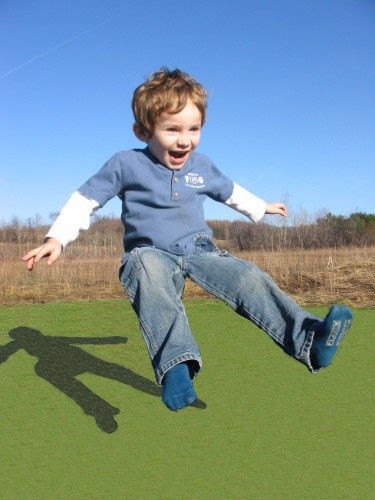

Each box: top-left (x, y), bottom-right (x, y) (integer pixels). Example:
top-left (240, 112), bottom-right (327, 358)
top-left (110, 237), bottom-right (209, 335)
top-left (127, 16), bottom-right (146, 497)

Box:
top-left (21, 66), bottom-right (354, 412)
